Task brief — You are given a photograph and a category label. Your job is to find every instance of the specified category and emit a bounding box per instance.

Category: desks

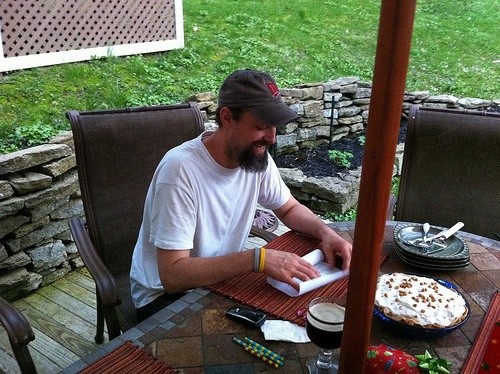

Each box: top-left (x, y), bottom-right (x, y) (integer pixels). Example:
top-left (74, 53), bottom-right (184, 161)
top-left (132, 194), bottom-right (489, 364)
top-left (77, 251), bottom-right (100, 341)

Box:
top-left (54, 217), bottom-right (499, 374)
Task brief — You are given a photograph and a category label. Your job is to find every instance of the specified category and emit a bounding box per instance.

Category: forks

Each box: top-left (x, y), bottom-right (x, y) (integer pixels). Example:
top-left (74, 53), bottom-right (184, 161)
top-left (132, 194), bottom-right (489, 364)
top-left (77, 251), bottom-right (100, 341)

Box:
top-left (419, 222), bottom-right (430, 253)
top-left (399, 230), bottom-right (444, 245)
top-left (430, 221), bottom-right (464, 254)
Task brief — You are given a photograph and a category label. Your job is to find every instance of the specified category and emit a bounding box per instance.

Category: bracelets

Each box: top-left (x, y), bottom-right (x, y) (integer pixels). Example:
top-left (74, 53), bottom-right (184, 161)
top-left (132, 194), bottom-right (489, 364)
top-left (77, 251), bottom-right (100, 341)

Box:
top-left (254, 247), bottom-right (265, 273)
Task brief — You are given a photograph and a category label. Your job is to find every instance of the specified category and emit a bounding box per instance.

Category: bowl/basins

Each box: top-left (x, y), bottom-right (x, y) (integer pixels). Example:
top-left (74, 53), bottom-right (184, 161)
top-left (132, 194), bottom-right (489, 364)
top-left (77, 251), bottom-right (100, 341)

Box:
top-left (374, 272), bottom-right (472, 340)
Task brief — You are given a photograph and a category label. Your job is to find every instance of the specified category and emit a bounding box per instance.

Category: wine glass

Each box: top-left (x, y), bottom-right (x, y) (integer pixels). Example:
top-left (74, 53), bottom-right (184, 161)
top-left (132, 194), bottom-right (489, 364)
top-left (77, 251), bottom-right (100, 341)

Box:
top-left (304, 298), bottom-right (345, 374)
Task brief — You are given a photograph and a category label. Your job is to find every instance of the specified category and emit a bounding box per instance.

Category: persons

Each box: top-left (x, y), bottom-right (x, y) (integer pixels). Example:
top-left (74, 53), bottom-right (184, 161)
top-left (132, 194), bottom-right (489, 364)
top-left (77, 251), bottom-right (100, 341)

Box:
top-left (130, 68), bottom-right (353, 323)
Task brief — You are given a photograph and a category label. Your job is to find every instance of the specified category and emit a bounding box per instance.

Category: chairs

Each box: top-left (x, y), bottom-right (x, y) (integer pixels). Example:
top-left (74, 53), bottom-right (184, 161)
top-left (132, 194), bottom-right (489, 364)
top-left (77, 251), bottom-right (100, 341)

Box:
top-left (65, 100), bottom-right (279, 343)
top-left (386, 104), bottom-right (500, 243)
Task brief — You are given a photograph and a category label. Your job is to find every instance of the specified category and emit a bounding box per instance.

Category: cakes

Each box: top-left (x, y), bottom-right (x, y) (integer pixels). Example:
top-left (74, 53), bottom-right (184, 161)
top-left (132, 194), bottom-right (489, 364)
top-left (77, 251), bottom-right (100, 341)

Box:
top-left (374, 272), bottom-right (468, 329)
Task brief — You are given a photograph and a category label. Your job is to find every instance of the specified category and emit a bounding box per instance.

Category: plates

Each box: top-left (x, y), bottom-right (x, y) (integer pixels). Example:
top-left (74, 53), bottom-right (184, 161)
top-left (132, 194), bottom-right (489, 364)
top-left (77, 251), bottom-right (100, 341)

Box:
top-left (394, 224), bottom-right (471, 274)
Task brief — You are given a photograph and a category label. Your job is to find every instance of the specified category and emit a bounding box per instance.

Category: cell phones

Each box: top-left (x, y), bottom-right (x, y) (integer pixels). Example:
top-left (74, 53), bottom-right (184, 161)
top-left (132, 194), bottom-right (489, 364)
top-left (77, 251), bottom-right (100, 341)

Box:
top-left (225, 304), bottom-right (267, 328)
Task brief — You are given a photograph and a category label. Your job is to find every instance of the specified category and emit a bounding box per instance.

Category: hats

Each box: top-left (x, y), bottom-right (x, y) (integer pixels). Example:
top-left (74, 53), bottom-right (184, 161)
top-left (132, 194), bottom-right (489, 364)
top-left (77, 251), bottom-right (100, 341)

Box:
top-left (218, 69), bottom-right (302, 127)
